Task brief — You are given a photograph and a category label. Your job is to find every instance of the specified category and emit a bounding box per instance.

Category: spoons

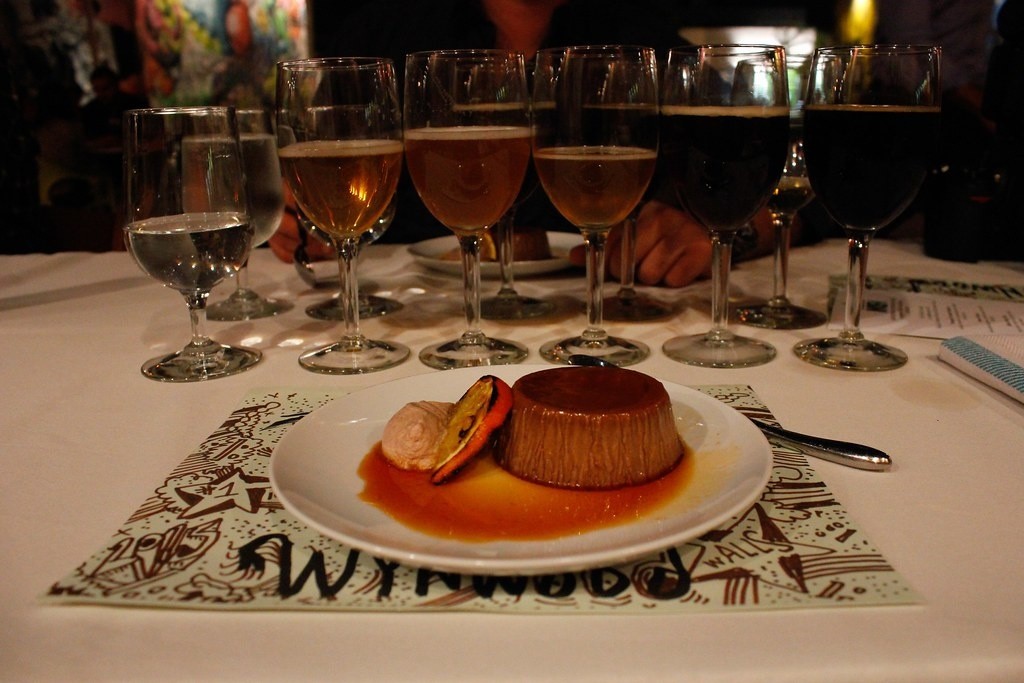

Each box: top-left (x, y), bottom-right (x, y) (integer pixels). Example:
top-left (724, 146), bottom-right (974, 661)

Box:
top-left (565, 354), bottom-right (893, 472)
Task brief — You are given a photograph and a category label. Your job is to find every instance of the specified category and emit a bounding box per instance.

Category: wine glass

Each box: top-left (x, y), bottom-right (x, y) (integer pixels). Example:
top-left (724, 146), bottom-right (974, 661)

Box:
top-left (304, 201), bottom-right (402, 321)
top-left (403, 48), bottom-right (529, 368)
top-left (461, 206), bottom-right (558, 320)
top-left (179, 107), bottom-right (295, 322)
top-left (729, 54), bottom-right (841, 331)
top-left (122, 106), bottom-right (263, 383)
top-left (792, 43), bottom-right (944, 372)
top-left (275, 56), bottom-right (410, 374)
top-left (579, 185), bottom-right (680, 324)
top-left (660, 44), bottom-right (789, 368)
top-left (530, 47), bottom-right (659, 369)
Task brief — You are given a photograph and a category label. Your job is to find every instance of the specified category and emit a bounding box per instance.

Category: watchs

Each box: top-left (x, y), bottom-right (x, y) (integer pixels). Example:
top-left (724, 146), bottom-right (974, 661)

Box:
top-left (732, 220), bottom-right (760, 263)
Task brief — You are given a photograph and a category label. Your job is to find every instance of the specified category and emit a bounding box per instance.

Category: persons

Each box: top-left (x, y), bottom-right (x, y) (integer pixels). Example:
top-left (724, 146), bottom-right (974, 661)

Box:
top-left (0, 0), bottom-right (1024, 287)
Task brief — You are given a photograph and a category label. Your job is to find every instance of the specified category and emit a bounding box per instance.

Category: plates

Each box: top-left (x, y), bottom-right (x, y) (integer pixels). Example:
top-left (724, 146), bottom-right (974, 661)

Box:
top-left (406, 231), bottom-right (586, 278)
top-left (270, 362), bottom-right (775, 575)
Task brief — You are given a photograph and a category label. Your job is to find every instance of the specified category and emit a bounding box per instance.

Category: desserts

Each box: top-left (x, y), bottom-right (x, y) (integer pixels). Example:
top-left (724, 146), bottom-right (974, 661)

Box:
top-left (495, 366), bottom-right (685, 490)
top-left (481, 226), bottom-right (552, 261)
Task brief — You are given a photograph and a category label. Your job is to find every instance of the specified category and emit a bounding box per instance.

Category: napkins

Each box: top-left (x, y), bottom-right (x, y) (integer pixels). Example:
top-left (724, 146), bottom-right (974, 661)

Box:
top-left (939, 334), bottom-right (1024, 404)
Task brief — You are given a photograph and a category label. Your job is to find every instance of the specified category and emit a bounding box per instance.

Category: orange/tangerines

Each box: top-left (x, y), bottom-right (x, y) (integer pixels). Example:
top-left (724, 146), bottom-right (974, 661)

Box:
top-left (432, 375), bottom-right (512, 486)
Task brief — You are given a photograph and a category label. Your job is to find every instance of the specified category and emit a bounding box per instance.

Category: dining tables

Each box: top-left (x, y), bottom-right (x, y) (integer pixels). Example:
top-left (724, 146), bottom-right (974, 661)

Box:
top-left (1, 237), bottom-right (1024, 683)
